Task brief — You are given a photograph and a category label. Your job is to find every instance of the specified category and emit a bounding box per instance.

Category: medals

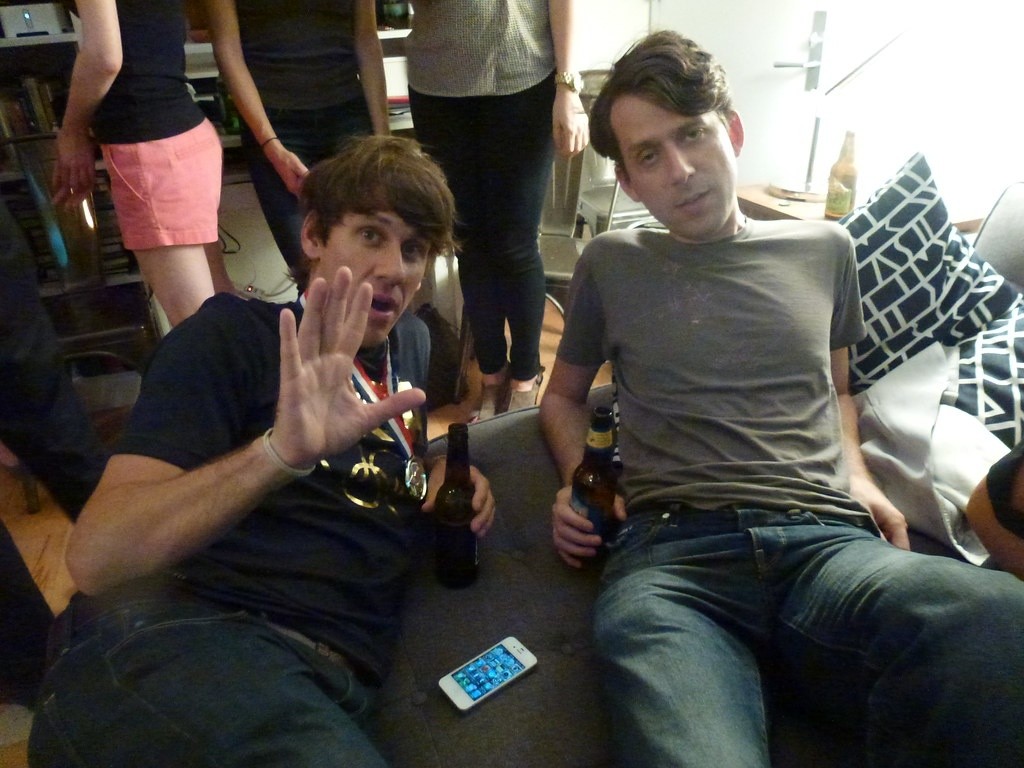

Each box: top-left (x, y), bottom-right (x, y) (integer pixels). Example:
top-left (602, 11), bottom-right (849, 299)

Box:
top-left (406, 459), bottom-right (427, 499)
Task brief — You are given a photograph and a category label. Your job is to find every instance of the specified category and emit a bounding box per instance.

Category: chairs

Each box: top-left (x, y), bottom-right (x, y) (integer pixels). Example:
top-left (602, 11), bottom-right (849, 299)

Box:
top-left (19, 353), bottom-right (143, 515)
top-left (449, 70), bottom-right (667, 414)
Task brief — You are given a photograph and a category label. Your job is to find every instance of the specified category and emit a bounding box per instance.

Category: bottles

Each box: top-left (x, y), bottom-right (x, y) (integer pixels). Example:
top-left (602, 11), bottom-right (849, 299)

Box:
top-left (822, 129), bottom-right (859, 222)
top-left (434, 421), bottom-right (478, 588)
top-left (216, 70), bottom-right (243, 135)
top-left (571, 404), bottom-right (617, 569)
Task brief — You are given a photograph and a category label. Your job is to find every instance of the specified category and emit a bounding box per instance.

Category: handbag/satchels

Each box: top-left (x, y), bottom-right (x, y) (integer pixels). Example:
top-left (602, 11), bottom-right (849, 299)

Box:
top-left (411, 303), bottom-right (470, 410)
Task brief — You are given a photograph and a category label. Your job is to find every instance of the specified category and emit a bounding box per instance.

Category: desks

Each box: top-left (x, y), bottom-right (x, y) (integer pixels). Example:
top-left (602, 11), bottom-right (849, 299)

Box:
top-left (737, 183), bottom-right (989, 232)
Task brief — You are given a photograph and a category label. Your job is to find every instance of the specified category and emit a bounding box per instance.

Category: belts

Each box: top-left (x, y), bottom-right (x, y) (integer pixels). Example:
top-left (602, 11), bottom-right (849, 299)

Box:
top-left (48, 580), bottom-right (367, 680)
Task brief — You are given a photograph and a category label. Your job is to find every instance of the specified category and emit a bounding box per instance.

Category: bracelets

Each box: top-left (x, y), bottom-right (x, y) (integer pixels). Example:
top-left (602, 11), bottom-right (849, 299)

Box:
top-left (264, 428), bottom-right (316, 476)
top-left (261, 137), bottom-right (278, 145)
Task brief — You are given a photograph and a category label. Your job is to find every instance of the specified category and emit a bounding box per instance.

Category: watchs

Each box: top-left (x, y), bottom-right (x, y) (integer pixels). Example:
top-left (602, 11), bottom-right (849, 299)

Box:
top-left (555, 72), bottom-right (583, 90)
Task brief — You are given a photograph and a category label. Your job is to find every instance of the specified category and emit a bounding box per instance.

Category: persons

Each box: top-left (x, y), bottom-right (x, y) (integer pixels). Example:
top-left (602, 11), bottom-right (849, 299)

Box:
top-left (543, 31), bottom-right (1024, 768)
top-left (47, 134), bottom-right (496, 768)
top-left (201, 0), bottom-right (390, 287)
top-left (0, 203), bottom-right (108, 714)
top-left (401, 0), bottom-right (589, 427)
top-left (967, 439), bottom-right (1024, 583)
top-left (50, 0), bottom-right (222, 325)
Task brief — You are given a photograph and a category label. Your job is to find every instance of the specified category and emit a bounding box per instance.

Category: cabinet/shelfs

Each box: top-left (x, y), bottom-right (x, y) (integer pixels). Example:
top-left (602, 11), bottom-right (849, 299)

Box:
top-left (0, 13), bottom-right (173, 351)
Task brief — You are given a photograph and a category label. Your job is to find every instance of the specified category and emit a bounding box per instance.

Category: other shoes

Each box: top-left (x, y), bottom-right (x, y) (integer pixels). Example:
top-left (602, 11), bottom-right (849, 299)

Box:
top-left (467, 361), bottom-right (510, 426)
top-left (498, 365), bottom-right (545, 416)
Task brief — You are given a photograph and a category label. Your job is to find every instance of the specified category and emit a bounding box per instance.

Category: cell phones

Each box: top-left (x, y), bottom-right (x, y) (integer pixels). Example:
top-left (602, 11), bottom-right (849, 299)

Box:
top-left (439, 636), bottom-right (538, 711)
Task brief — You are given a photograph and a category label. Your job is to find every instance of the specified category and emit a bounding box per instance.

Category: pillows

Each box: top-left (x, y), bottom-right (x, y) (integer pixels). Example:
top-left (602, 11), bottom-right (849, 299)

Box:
top-left (838, 151), bottom-right (1024, 450)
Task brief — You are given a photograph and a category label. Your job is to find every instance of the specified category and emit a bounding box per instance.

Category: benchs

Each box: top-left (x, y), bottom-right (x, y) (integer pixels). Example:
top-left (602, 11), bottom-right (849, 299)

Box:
top-left (372, 184), bottom-right (1024, 768)
